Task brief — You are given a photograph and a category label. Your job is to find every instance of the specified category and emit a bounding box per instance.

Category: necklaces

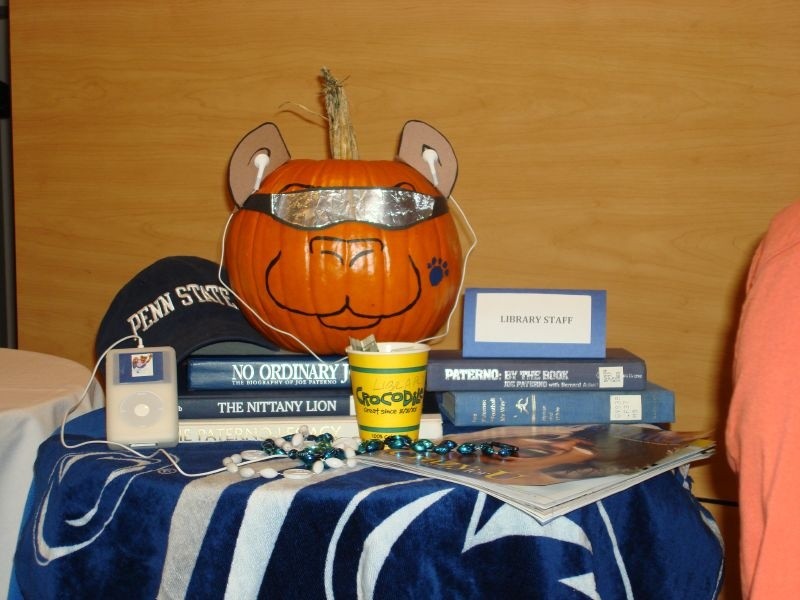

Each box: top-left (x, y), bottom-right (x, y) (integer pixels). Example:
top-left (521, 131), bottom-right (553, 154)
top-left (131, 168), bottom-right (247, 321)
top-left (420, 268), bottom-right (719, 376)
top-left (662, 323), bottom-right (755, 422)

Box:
top-left (222, 424), bottom-right (519, 480)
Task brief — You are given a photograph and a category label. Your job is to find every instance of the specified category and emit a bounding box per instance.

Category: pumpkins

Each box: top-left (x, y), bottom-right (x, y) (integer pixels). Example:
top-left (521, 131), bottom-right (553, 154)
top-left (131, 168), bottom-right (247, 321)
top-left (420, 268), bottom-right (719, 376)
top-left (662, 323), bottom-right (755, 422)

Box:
top-left (224, 70), bottom-right (463, 355)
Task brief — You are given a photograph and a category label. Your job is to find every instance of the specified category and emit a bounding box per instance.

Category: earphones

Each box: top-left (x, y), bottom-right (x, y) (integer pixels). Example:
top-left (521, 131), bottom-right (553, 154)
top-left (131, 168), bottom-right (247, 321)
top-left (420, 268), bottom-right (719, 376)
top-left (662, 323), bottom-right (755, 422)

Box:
top-left (423, 148), bottom-right (439, 187)
top-left (254, 154), bottom-right (269, 188)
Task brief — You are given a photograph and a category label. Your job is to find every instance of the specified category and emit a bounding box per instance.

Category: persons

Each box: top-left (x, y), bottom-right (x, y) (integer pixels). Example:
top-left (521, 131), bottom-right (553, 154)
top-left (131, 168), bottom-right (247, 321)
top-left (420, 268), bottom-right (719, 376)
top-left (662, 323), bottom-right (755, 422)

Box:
top-left (433, 428), bottom-right (709, 486)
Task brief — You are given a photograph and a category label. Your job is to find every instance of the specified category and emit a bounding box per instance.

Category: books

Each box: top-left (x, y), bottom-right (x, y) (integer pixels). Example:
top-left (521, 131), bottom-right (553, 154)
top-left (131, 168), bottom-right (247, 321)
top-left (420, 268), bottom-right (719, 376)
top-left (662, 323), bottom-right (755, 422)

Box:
top-left (426, 346), bottom-right (647, 392)
top-left (173, 354), bottom-right (362, 443)
top-left (434, 380), bottom-right (676, 428)
top-left (355, 420), bottom-right (717, 527)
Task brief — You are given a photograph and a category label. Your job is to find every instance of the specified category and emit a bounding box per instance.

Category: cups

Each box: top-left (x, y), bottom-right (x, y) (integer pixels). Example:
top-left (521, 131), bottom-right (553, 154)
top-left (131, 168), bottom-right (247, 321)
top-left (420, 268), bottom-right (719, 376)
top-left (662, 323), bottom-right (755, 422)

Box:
top-left (345, 342), bottom-right (432, 451)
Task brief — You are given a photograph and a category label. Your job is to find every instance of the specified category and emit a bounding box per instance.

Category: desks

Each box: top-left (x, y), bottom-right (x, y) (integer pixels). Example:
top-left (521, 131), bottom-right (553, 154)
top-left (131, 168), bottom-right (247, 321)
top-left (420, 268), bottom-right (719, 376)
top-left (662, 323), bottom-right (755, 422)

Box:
top-left (8, 407), bottom-right (725, 600)
top-left (0, 348), bottom-right (105, 600)
